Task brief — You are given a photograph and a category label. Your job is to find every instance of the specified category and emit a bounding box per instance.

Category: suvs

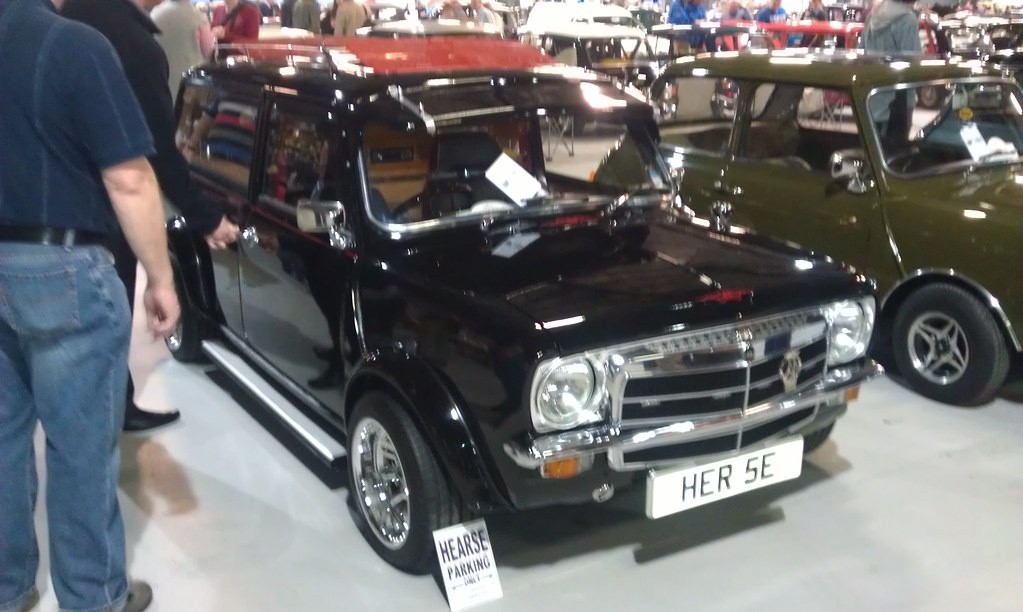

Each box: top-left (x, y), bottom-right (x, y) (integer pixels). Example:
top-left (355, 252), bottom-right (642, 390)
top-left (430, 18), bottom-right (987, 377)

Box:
top-left (645, 44), bottom-right (1022, 408)
top-left (148, 32), bottom-right (888, 573)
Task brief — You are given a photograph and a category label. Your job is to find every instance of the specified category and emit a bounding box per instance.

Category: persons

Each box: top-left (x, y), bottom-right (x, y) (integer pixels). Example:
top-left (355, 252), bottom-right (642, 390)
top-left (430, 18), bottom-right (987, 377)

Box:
top-left (666, 0), bottom-right (830, 55)
top-left (259, 0), bottom-right (515, 37)
top-left (863, 0), bottom-right (922, 140)
top-left (277, 140), bottom-right (350, 390)
top-left (183, 80), bottom-right (257, 185)
top-left (1, 0), bottom-right (260, 612)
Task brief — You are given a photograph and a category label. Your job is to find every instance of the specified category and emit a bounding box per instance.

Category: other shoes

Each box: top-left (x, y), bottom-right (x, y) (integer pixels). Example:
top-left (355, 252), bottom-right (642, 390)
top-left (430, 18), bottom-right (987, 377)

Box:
top-left (21, 588), bottom-right (40, 612)
top-left (120, 579), bottom-right (152, 612)
top-left (123, 403), bottom-right (179, 430)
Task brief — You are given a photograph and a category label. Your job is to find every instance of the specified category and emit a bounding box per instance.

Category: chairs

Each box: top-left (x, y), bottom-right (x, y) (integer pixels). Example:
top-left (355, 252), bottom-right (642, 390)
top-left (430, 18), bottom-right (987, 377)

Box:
top-left (285, 184), bottom-right (391, 224)
top-left (423, 133), bottom-right (503, 220)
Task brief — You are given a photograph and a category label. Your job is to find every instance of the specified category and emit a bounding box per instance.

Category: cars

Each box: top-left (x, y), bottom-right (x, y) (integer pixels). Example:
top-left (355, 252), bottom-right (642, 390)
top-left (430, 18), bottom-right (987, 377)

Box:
top-left (359, 1), bottom-right (1023, 134)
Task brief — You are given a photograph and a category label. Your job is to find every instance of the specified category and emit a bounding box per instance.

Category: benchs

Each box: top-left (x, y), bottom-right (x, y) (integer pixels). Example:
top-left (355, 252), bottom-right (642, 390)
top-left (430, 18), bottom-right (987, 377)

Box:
top-left (660, 120), bottom-right (802, 158)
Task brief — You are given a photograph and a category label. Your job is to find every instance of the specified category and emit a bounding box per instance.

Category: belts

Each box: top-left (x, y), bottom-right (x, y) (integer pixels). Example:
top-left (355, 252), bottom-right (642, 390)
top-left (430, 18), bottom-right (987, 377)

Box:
top-left (0, 225), bottom-right (95, 244)
